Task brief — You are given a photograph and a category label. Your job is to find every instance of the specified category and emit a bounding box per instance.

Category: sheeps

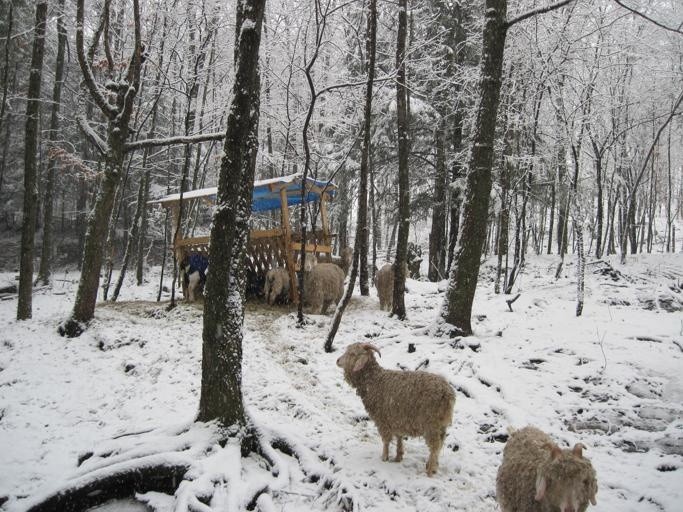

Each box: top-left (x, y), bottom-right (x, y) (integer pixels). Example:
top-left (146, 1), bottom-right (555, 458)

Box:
top-left (373, 260), bottom-right (409, 311)
top-left (180, 251), bottom-right (208, 303)
top-left (263, 246), bottom-right (354, 315)
top-left (496, 426), bottom-right (598, 512)
top-left (336, 342), bottom-right (456, 477)
